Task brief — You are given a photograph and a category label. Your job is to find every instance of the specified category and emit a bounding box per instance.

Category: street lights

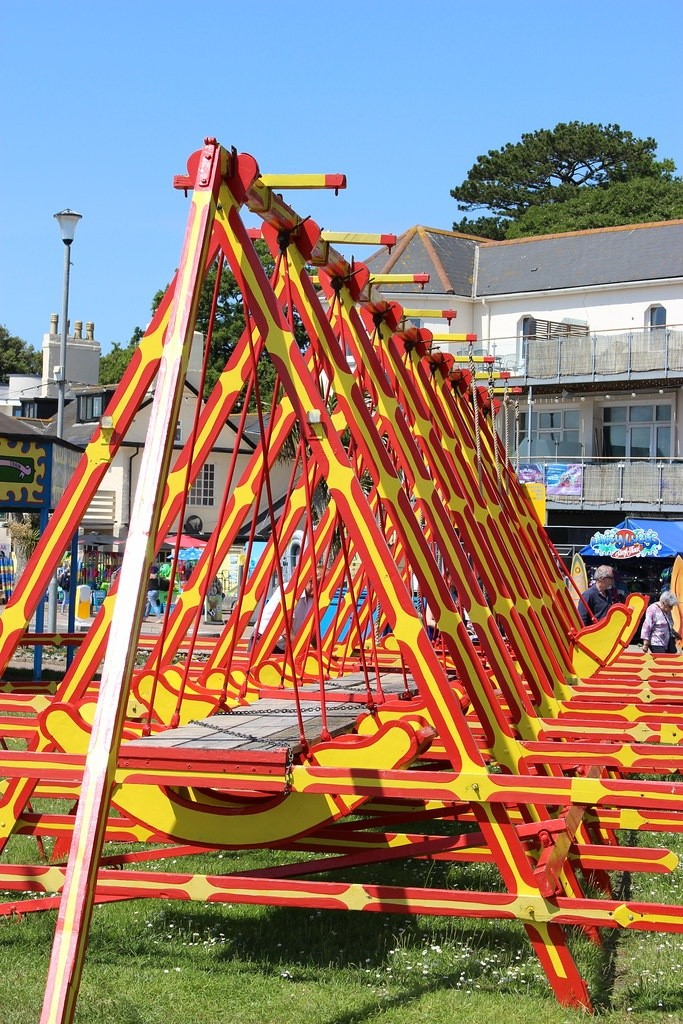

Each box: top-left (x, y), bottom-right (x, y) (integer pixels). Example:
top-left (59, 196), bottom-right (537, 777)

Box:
top-left (45, 206), bottom-right (84, 633)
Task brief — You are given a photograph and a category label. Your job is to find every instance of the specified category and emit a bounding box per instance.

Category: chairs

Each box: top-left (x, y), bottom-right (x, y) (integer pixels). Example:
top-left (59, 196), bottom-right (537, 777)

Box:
top-left (457, 349), bottom-right (519, 377)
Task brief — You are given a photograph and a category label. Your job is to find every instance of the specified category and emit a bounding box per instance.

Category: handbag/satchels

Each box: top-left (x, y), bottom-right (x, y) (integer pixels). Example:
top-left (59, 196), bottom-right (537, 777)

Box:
top-left (665, 636), bottom-right (677, 653)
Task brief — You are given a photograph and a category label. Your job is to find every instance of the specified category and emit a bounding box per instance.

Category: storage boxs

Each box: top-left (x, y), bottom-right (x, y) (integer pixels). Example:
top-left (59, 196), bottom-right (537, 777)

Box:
top-left (162, 603), bottom-right (176, 614)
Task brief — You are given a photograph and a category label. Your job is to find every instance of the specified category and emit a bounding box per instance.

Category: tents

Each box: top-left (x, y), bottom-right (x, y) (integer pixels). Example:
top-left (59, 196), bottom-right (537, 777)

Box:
top-left (569, 516), bottom-right (683, 648)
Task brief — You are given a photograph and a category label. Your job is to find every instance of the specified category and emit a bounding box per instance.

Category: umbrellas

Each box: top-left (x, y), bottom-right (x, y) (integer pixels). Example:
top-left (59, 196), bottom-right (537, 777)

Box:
top-left (70, 531), bottom-right (125, 563)
top-left (162, 534), bottom-right (208, 566)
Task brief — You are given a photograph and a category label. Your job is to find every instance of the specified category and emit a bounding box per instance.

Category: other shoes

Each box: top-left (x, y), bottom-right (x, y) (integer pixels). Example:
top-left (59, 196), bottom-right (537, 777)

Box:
top-left (156, 620), bottom-right (162, 624)
top-left (142, 617), bottom-right (146, 621)
top-left (61, 612), bottom-right (66, 615)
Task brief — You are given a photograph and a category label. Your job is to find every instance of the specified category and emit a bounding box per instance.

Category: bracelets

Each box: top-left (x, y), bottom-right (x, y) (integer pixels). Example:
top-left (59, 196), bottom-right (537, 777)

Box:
top-left (673, 631), bottom-right (676, 634)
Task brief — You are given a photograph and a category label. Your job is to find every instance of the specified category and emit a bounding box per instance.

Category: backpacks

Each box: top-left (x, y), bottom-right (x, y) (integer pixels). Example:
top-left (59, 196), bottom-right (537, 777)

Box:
top-left (62, 571), bottom-right (71, 590)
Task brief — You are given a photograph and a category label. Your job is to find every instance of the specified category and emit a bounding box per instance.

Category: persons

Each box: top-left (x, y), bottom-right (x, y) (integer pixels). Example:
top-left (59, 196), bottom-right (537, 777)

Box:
top-left (578, 565), bottom-right (614, 627)
top-left (641, 591), bottom-right (682, 654)
top-left (247, 565), bottom-right (339, 662)
top-left (142, 566), bottom-right (162, 624)
top-left (82, 566), bottom-right (116, 585)
top-left (165, 560), bottom-right (194, 581)
top-left (426, 568), bottom-right (464, 645)
top-left (61, 560), bottom-right (71, 616)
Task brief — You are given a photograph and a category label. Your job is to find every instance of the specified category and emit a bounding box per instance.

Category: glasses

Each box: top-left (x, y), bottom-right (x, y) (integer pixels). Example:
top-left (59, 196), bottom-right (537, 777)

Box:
top-left (604, 576), bottom-right (614, 580)
